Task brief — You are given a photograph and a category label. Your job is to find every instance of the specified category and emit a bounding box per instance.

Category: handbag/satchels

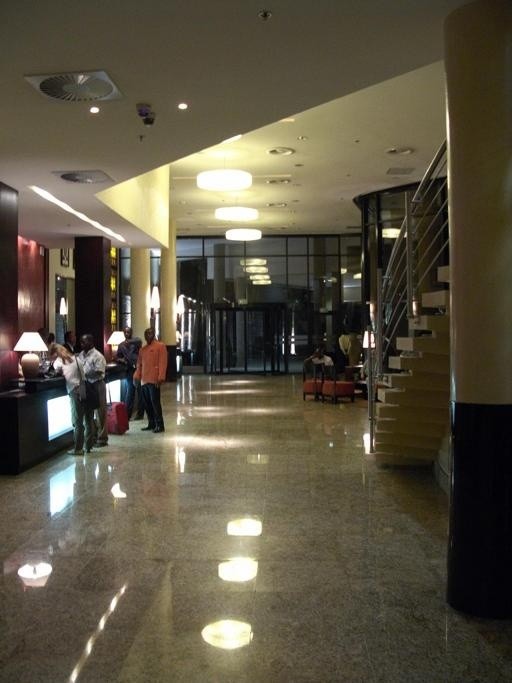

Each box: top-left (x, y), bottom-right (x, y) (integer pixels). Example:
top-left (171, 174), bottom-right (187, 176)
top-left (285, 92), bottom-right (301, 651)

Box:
top-left (76, 379), bottom-right (100, 409)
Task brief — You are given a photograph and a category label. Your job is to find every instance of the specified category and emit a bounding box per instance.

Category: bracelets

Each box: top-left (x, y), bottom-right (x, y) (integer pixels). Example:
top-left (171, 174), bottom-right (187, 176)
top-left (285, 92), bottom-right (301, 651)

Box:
top-left (311, 356), bottom-right (313, 359)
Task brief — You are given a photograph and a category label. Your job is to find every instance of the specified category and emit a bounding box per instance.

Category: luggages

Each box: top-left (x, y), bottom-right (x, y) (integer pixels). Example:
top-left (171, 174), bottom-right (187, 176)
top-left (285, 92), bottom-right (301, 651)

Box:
top-left (103, 371), bottom-right (129, 434)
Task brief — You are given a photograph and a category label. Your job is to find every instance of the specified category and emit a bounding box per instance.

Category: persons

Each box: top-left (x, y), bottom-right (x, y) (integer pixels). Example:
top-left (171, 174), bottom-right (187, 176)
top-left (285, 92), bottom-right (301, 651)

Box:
top-left (339, 330), bottom-right (352, 366)
top-left (50, 343), bottom-right (96, 455)
top-left (133, 328), bottom-right (168, 433)
top-left (304, 348), bottom-right (334, 369)
top-left (113, 327), bottom-right (144, 420)
top-left (62, 331), bottom-right (77, 355)
top-left (18, 328), bottom-right (50, 380)
top-left (77, 334), bottom-right (108, 448)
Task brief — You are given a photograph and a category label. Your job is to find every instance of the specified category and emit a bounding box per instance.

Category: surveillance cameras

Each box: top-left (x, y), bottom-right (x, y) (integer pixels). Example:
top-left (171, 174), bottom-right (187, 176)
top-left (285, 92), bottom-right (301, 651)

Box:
top-left (143, 115), bottom-right (155, 125)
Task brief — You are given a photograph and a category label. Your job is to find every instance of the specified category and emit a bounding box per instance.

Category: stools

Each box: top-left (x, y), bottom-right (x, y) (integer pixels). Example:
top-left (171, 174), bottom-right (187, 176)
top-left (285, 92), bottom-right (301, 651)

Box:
top-left (299, 364), bottom-right (332, 403)
top-left (320, 368), bottom-right (354, 403)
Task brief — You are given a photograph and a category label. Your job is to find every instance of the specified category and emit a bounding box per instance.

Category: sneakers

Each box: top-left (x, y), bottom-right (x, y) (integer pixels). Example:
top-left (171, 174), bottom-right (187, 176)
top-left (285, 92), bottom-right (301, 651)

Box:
top-left (66, 440), bottom-right (110, 455)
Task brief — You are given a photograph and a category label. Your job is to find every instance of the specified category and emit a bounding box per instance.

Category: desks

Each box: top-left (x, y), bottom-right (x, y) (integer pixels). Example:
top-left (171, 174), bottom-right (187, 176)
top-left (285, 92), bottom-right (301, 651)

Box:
top-left (353, 379), bottom-right (369, 400)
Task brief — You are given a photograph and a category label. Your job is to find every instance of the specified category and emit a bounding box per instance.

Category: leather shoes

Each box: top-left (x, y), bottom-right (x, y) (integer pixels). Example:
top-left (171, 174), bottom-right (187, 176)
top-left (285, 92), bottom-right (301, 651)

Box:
top-left (133, 415), bottom-right (143, 420)
top-left (152, 427), bottom-right (164, 432)
top-left (140, 425), bottom-right (154, 431)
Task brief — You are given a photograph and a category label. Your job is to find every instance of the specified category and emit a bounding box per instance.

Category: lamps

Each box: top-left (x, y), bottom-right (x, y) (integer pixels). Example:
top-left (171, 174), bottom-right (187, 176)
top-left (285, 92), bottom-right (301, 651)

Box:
top-left (196, 168), bottom-right (265, 242)
top-left (361, 329), bottom-right (378, 349)
top-left (238, 257), bottom-right (275, 286)
top-left (105, 328), bottom-right (129, 363)
top-left (10, 329), bottom-right (52, 380)
